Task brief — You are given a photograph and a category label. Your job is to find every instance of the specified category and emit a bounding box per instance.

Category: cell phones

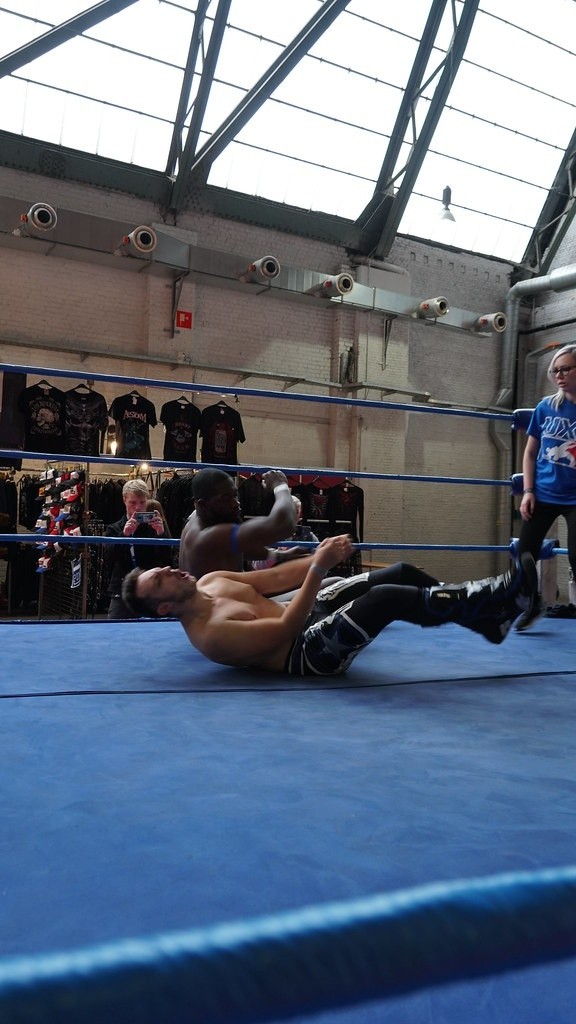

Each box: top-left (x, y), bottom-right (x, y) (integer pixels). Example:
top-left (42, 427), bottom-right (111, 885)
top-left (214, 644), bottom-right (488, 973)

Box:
top-left (134, 512), bottom-right (157, 524)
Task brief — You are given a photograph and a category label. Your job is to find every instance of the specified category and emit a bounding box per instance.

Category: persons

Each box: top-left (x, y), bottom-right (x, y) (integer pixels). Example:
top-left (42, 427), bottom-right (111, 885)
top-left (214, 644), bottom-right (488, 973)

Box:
top-left (510, 343), bottom-right (576, 634)
top-left (179, 466), bottom-right (347, 602)
top-left (146, 499), bottom-right (172, 539)
top-left (122, 532), bottom-right (537, 678)
top-left (104, 479), bottom-right (173, 619)
top-left (252, 494), bottom-right (321, 572)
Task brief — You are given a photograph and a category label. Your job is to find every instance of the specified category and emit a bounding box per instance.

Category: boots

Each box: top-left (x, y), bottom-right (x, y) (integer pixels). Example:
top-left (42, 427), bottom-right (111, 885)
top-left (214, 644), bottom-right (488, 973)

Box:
top-left (419, 554), bottom-right (539, 645)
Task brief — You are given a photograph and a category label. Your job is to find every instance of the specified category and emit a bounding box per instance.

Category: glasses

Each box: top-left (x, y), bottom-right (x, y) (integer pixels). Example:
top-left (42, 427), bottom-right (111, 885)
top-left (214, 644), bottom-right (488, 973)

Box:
top-left (551, 365), bottom-right (576, 376)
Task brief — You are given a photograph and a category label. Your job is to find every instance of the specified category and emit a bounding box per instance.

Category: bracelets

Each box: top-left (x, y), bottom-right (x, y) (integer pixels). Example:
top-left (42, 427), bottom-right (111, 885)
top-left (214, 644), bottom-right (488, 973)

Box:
top-left (273, 484), bottom-right (290, 493)
top-left (524, 488), bottom-right (534, 494)
top-left (310, 563), bottom-right (329, 576)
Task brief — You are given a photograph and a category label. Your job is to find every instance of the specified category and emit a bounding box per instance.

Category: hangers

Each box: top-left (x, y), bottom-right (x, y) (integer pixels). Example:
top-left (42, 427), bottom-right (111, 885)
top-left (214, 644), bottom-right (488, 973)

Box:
top-left (18, 372), bottom-right (365, 559)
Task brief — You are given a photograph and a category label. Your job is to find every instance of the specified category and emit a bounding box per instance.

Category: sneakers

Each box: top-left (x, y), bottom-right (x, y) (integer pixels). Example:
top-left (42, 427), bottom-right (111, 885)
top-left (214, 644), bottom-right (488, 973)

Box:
top-left (513, 593), bottom-right (546, 632)
